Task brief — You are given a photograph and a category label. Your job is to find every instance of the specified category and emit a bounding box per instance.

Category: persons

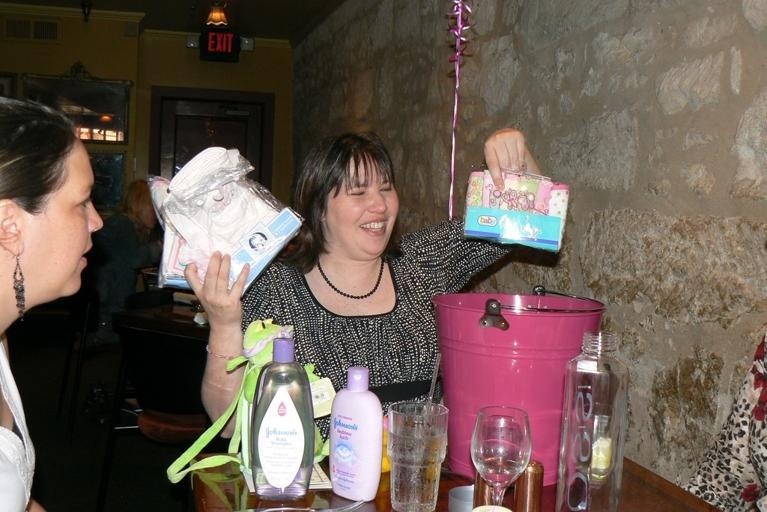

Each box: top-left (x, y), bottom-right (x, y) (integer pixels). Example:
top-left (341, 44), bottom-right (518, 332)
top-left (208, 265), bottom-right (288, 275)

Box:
top-left (183, 126), bottom-right (542, 439)
top-left (0, 97), bottom-right (104, 512)
top-left (95, 180), bottom-right (162, 430)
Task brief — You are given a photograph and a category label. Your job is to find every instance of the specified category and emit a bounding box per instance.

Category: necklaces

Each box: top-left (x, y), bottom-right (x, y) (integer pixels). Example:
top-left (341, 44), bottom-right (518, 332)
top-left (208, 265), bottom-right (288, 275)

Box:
top-left (316, 255), bottom-right (385, 299)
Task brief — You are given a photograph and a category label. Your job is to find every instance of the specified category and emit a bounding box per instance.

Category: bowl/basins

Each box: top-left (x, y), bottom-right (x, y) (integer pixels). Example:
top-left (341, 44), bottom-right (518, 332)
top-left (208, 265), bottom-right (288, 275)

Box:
top-left (448, 486), bottom-right (472, 510)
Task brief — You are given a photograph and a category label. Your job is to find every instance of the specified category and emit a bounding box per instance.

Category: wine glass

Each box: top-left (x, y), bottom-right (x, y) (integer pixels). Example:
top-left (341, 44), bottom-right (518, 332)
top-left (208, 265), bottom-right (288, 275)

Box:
top-left (463, 403), bottom-right (532, 512)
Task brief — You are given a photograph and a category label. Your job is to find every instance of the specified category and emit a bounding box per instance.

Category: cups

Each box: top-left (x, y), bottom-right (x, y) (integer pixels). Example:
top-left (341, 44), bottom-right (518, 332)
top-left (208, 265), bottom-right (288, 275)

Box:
top-left (389, 400), bottom-right (448, 511)
top-left (573, 397), bottom-right (617, 488)
top-left (469, 505), bottom-right (512, 512)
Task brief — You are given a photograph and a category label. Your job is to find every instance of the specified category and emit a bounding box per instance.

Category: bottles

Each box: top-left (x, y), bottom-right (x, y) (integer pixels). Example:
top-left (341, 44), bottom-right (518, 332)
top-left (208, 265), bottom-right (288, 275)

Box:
top-left (553, 331), bottom-right (625, 511)
top-left (513, 461), bottom-right (544, 511)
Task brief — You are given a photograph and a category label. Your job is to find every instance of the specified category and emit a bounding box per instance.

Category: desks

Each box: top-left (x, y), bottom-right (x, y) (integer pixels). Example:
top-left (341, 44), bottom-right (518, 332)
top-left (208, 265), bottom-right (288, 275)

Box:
top-left (189, 450), bottom-right (719, 512)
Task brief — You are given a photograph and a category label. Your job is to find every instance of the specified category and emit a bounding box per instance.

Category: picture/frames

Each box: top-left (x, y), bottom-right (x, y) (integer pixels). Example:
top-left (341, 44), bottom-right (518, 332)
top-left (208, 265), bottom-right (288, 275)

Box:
top-left (87, 149), bottom-right (133, 215)
top-left (0, 71), bottom-right (18, 99)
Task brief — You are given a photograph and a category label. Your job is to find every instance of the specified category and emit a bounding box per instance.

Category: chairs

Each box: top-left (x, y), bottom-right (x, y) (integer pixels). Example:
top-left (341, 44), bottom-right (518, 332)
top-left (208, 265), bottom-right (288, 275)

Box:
top-left (96, 312), bottom-right (210, 512)
top-left (58, 287), bottom-right (121, 448)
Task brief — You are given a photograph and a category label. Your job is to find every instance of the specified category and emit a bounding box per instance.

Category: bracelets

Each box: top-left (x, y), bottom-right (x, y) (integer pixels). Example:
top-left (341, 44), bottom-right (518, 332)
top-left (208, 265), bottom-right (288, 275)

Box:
top-left (206, 344), bottom-right (244, 359)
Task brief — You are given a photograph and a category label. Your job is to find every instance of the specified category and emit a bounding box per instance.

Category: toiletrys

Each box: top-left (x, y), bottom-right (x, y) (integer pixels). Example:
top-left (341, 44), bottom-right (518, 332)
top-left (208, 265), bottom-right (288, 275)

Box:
top-left (328, 367), bottom-right (384, 503)
top-left (250, 338), bottom-right (315, 502)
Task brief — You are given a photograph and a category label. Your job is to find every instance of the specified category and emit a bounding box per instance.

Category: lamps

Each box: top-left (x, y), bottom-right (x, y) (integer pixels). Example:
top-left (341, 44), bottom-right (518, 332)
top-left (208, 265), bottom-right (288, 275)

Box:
top-left (204, 0), bottom-right (229, 26)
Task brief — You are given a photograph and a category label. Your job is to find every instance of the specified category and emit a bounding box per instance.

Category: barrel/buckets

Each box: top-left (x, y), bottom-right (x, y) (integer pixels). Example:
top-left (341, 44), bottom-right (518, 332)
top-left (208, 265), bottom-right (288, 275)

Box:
top-left (429, 285), bottom-right (608, 489)
